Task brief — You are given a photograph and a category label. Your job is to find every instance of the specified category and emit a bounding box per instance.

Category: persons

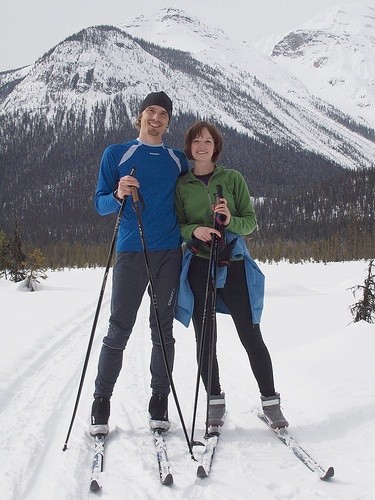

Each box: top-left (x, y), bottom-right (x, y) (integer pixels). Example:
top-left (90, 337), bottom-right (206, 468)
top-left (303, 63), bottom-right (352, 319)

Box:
top-left (173, 121), bottom-right (290, 428)
top-left (89, 90), bottom-right (189, 435)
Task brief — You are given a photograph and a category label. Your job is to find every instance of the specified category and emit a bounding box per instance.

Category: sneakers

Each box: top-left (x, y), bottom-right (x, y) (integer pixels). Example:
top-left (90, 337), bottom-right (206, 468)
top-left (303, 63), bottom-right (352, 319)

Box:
top-left (260, 393), bottom-right (289, 429)
top-left (88, 399), bottom-right (110, 437)
top-left (205, 390), bottom-right (226, 436)
top-left (147, 394), bottom-right (171, 430)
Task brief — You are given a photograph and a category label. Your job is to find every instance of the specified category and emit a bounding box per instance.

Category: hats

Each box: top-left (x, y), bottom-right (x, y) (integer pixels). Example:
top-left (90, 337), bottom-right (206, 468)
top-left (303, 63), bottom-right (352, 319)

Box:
top-left (140, 91), bottom-right (173, 118)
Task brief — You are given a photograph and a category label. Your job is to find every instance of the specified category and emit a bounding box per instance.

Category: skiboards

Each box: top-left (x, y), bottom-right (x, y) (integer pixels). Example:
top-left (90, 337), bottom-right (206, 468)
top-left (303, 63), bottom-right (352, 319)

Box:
top-left (197, 408), bottom-right (335, 478)
top-left (89, 428), bottom-right (174, 493)
